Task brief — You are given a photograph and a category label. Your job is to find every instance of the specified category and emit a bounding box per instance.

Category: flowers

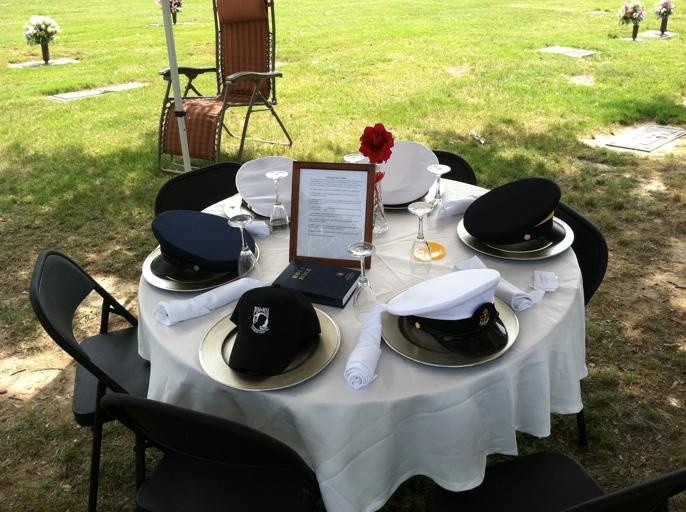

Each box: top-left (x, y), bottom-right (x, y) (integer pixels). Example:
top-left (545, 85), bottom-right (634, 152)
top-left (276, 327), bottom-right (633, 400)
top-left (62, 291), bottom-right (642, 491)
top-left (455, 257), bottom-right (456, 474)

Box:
top-left (358, 123), bottom-right (394, 232)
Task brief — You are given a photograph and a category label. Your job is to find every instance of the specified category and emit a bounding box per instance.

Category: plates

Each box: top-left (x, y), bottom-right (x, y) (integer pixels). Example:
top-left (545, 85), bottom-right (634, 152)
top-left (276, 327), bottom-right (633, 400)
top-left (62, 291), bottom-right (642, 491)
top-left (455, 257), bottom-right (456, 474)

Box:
top-left (380, 290), bottom-right (519, 368)
top-left (221, 198), bottom-right (257, 224)
top-left (456, 213), bottom-right (576, 262)
top-left (142, 242), bottom-right (260, 293)
top-left (385, 200), bottom-right (428, 215)
top-left (199, 303), bottom-right (342, 392)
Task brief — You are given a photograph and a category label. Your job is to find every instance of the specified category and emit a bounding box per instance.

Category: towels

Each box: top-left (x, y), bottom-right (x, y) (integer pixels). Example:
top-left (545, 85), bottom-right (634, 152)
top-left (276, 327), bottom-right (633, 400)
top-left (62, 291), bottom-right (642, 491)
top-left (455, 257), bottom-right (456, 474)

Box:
top-left (245, 219), bottom-right (270, 237)
top-left (343, 303), bottom-right (386, 389)
top-left (437, 194), bottom-right (481, 218)
top-left (456, 253), bottom-right (534, 312)
top-left (153, 277), bottom-right (265, 326)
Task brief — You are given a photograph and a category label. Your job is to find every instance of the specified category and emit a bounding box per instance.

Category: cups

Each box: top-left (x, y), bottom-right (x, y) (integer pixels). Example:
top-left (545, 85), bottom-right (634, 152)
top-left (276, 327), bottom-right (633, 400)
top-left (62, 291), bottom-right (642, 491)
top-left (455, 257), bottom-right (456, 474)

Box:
top-left (427, 164), bottom-right (451, 230)
top-left (348, 243), bottom-right (377, 324)
top-left (266, 171), bottom-right (291, 238)
top-left (228, 213), bottom-right (262, 282)
top-left (409, 201), bottom-right (435, 277)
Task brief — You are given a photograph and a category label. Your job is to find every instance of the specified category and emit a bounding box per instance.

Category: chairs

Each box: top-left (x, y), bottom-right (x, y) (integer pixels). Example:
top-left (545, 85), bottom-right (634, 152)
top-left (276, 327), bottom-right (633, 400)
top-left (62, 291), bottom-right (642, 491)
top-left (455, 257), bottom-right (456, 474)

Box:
top-left (30, 249), bottom-right (195, 512)
top-left (153, 161), bottom-right (245, 211)
top-left (423, 452), bottom-right (686, 508)
top-left (157, 1), bottom-right (294, 174)
top-left (556, 203), bottom-right (609, 307)
top-left (430, 148), bottom-right (477, 189)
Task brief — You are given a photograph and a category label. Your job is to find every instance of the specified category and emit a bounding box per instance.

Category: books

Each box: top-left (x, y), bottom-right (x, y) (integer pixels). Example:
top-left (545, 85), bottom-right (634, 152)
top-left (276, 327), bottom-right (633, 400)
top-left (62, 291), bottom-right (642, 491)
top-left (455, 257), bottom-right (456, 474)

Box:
top-left (270, 258), bottom-right (361, 309)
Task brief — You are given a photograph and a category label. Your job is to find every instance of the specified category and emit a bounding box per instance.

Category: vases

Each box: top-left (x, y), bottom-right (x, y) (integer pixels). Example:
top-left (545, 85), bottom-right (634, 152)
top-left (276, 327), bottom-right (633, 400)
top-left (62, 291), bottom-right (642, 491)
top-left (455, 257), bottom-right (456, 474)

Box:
top-left (618, 1), bottom-right (645, 41)
top-left (159, 1), bottom-right (183, 24)
top-left (655, 1), bottom-right (675, 37)
top-left (22, 13), bottom-right (62, 64)
top-left (372, 168), bottom-right (390, 242)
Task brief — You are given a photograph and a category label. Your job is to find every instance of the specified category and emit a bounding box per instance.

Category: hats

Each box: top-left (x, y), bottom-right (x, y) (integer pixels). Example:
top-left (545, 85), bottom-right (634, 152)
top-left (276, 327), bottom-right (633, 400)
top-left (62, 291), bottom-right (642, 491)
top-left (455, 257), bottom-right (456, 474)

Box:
top-left (385, 268), bottom-right (508, 360)
top-left (358, 139), bottom-right (439, 208)
top-left (150, 209), bottom-right (255, 285)
top-left (235, 156), bottom-right (297, 218)
top-left (228, 283), bottom-right (322, 376)
top-left (463, 177), bottom-right (566, 254)
